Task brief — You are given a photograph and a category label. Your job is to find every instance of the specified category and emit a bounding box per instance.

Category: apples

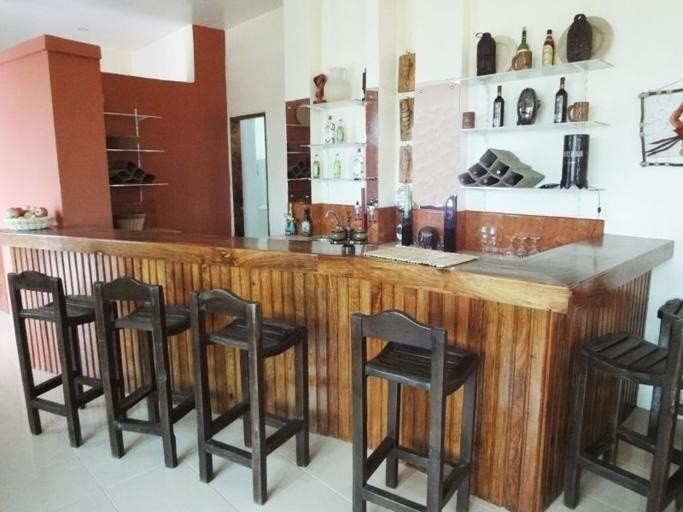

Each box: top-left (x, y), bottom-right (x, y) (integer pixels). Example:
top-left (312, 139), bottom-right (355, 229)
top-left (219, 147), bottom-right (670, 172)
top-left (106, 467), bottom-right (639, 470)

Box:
top-left (6, 205), bottom-right (48, 218)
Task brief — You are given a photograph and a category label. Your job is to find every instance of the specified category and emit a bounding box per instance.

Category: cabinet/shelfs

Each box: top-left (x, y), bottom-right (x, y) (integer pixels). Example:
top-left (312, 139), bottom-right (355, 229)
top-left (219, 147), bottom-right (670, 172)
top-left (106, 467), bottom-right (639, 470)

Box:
top-left (285, 88), bottom-right (379, 233)
top-left (461, 59), bottom-right (614, 191)
top-left (103, 108), bottom-right (169, 232)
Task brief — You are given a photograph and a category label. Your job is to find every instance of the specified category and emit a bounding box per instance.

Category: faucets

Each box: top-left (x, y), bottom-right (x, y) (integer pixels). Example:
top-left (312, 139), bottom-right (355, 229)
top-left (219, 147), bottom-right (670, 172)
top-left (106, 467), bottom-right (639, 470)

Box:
top-left (324, 211), bottom-right (346, 238)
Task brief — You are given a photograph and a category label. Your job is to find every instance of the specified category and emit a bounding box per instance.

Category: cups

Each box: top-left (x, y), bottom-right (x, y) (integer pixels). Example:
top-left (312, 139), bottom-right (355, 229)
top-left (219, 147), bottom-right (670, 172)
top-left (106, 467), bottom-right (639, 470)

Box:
top-left (567, 102), bottom-right (589, 122)
top-left (461, 111), bottom-right (475, 129)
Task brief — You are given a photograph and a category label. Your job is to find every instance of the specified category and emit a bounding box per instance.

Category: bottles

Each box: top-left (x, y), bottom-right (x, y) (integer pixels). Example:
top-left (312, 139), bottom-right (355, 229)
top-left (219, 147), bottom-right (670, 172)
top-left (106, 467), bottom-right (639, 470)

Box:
top-left (554, 77), bottom-right (567, 123)
top-left (312, 148), bottom-right (364, 179)
top-left (335, 117), bottom-right (344, 143)
top-left (301, 208), bottom-right (313, 237)
top-left (324, 114), bottom-right (335, 144)
top-left (476, 13), bottom-right (591, 76)
top-left (459, 168), bottom-right (518, 185)
top-left (492, 85), bottom-right (504, 126)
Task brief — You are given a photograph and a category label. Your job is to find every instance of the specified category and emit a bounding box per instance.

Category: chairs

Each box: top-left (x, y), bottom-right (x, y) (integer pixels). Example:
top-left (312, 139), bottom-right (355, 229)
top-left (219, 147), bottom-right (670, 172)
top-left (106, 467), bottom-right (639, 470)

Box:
top-left (563, 297), bottom-right (682, 511)
top-left (93, 276), bottom-right (191, 468)
top-left (350, 310), bottom-right (479, 512)
top-left (188, 288), bottom-right (311, 505)
top-left (7, 270), bottom-right (128, 448)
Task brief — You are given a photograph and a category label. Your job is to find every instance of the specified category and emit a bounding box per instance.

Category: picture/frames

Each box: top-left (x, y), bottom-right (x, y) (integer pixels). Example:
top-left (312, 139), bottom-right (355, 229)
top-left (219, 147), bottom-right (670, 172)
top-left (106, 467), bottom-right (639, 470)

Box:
top-left (639, 89), bottom-right (683, 167)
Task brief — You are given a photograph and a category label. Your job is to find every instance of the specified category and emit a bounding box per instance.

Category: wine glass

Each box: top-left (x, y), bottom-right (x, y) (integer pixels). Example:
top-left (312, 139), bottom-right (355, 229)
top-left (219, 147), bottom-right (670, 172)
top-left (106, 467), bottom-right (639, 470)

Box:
top-left (480, 223), bottom-right (542, 258)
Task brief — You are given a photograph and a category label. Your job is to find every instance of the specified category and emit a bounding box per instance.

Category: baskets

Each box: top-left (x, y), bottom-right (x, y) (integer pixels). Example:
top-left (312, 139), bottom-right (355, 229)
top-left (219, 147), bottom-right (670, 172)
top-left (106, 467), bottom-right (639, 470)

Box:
top-left (114, 211), bottom-right (147, 230)
top-left (4, 215), bottom-right (54, 230)
